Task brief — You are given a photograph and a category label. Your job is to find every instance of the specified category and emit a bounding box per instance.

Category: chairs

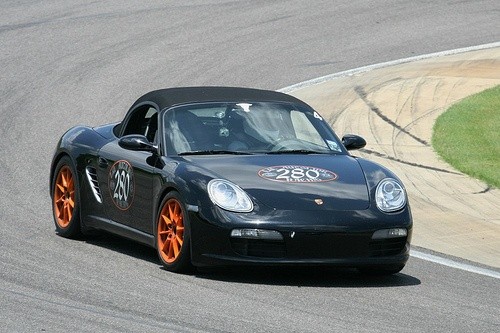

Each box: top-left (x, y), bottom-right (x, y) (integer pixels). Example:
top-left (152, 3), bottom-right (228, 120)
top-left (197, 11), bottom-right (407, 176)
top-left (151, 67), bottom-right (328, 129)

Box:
top-left (165, 109), bottom-right (214, 150)
top-left (145, 112), bottom-right (159, 142)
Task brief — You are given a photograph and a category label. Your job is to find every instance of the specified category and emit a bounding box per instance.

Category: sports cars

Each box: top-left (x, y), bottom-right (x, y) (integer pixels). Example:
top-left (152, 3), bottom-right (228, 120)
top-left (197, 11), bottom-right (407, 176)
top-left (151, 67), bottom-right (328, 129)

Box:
top-left (48, 85), bottom-right (414, 273)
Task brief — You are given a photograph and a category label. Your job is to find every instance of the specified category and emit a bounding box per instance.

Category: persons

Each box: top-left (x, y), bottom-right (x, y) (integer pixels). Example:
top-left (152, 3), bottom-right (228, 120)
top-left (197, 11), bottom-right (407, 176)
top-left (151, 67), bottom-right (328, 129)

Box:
top-left (228, 104), bottom-right (283, 154)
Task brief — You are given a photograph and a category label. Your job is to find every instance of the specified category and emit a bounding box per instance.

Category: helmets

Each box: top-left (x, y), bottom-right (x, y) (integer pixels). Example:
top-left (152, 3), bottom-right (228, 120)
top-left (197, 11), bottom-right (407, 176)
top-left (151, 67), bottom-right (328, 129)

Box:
top-left (243, 107), bottom-right (282, 146)
